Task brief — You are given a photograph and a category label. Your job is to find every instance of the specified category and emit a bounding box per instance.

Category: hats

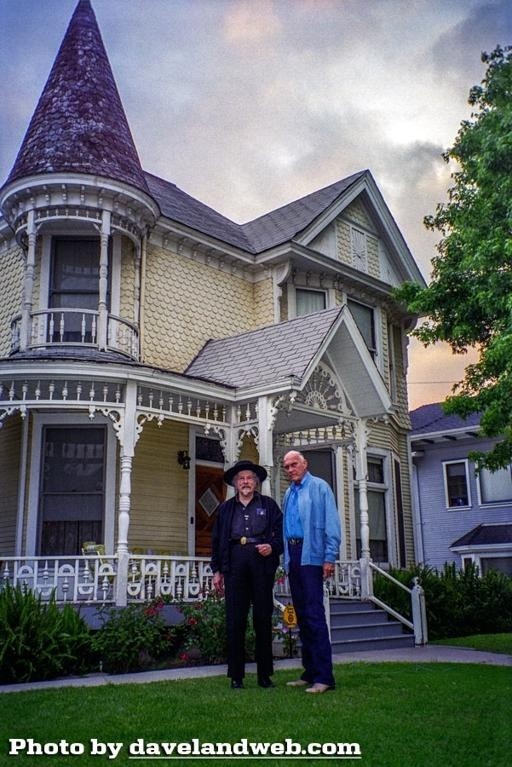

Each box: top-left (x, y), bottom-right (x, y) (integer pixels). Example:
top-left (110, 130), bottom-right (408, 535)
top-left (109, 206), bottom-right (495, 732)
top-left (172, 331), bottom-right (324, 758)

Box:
top-left (221, 459), bottom-right (267, 486)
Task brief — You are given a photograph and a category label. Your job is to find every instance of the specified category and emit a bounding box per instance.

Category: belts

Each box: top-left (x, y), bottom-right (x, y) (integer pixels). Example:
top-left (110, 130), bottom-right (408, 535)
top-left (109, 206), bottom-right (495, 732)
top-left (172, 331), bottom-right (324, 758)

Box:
top-left (287, 536), bottom-right (303, 545)
top-left (229, 536), bottom-right (265, 545)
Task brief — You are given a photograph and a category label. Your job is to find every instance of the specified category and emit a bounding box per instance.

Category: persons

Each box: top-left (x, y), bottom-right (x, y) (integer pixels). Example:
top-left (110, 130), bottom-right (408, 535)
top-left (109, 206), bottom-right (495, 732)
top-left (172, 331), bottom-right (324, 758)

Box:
top-left (283, 449), bottom-right (344, 693)
top-left (209, 459), bottom-right (283, 688)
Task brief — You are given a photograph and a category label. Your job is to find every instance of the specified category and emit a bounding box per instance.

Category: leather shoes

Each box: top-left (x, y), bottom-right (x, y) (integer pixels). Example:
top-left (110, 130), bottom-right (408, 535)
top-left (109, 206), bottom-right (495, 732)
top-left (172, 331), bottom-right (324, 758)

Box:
top-left (256, 676), bottom-right (276, 690)
top-left (230, 679), bottom-right (245, 691)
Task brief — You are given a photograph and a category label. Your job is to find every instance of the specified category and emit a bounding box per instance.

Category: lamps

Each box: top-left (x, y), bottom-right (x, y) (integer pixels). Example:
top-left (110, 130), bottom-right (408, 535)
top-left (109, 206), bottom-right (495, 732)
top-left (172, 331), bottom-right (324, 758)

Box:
top-left (177, 449), bottom-right (192, 470)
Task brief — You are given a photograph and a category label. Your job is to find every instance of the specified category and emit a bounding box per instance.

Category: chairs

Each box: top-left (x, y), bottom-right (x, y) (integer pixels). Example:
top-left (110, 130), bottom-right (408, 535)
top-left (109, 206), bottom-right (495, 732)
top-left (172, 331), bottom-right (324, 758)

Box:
top-left (80, 541), bottom-right (138, 602)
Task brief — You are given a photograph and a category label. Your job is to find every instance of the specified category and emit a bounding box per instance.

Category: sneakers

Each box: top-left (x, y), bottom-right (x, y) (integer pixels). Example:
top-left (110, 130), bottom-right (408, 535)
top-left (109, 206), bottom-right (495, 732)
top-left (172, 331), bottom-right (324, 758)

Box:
top-left (285, 678), bottom-right (310, 686)
top-left (306, 682), bottom-right (334, 695)
